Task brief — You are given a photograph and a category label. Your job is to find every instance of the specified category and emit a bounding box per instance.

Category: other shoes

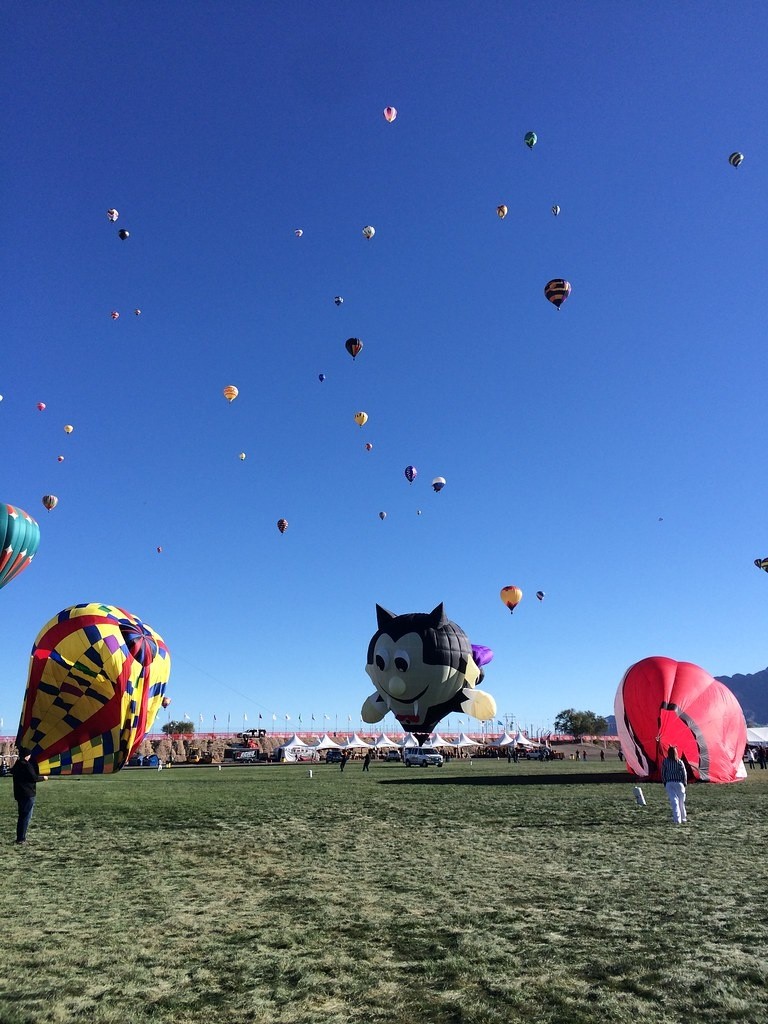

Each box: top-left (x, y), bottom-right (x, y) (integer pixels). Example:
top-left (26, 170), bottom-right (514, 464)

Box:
top-left (621, 761), bottom-right (623, 762)
top-left (750, 767), bottom-right (752, 769)
top-left (518, 761), bottom-right (520, 763)
top-left (604, 761), bottom-right (606, 762)
top-left (362, 770), bottom-right (365, 771)
top-left (753, 767), bottom-right (755, 769)
top-left (601, 760), bottom-right (602, 762)
top-left (367, 770), bottom-right (369, 771)
top-left (673, 819), bottom-right (688, 823)
top-left (158, 770), bottom-right (162, 772)
top-left (508, 761), bottom-right (510, 763)
top-left (760, 767), bottom-right (764, 769)
top-left (578, 760), bottom-right (580, 761)
top-left (584, 760), bottom-right (587, 762)
top-left (765, 767), bottom-right (767, 769)
top-left (576, 760), bottom-right (577, 761)
top-left (340, 769), bottom-right (344, 771)
top-left (16, 838), bottom-right (25, 843)
top-left (514, 761), bottom-right (517, 763)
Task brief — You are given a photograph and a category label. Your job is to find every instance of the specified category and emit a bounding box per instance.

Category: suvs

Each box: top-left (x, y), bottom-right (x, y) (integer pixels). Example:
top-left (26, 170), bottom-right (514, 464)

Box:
top-left (405, 747), bottom-right (443, 767)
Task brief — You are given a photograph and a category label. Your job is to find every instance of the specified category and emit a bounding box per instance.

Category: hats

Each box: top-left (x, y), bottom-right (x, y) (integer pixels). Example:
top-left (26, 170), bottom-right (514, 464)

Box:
top-left (18, 749), bottom-right (29, 756)
top-left (342, 750), bottom-right (347, 753)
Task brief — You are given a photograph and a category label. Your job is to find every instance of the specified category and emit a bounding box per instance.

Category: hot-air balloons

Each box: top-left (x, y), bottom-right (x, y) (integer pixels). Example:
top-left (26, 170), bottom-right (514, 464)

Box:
top-left (277, 519), bottom-right (289, 535)
top-left (361, 602), bottom-right (496, 747)
top-left (320, 335), bottom-right (373, 453)
top-left (613, 656), bottom-right (747, 784)
top-left (499, 584), bottom-right (545, 614)
top-left (42, 495), bottom-right (58, 514)
top-left (223, 384), bottom-right (246, 460)
top-left (107, 208), bottom-right (141, 321)
top-left (15, 601), bottom-right (172, 776)
top-left (294, 104), bottom-right (744, 312)
top-left (0, 394), bottom-right (74, 463)
top-left (380, 465), bottom-right (447, 521)
top-left (754, 557), bottom-right (768, 573)
top-left (0, 502), bottom-right (40, 590)
top-left (157, 546), bottom-right (162, 553)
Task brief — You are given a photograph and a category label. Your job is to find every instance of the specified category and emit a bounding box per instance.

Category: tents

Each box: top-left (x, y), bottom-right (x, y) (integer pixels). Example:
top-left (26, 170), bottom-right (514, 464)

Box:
top-left (273, 732), bottom-right (537, 763)
top-left (746, 727), bottom-right (768, 746)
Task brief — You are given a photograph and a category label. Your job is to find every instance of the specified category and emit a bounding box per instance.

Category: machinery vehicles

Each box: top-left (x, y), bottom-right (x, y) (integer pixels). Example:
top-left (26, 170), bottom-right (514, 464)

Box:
top-left (187, 747), bottom-right (214, 764)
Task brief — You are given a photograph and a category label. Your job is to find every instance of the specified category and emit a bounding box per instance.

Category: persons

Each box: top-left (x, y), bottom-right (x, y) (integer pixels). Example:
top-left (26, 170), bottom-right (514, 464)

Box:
top-left (9, 747), bottom-right (47, 844)
top-left (661, 744), bottom-right (687, 824)
top-left (745, 744), bottom-right (768, 769)
top-left (1, 761), bottom-right (9, 777)
top-left (122, 745), bottom-right (626, 772)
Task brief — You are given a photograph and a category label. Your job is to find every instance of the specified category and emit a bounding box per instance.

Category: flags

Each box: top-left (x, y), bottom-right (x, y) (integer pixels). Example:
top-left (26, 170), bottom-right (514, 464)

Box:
top-left (156, 713), bottom-right (504, 726)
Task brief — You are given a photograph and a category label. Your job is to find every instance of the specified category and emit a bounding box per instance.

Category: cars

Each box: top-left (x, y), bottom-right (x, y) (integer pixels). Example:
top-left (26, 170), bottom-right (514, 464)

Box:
top-left (238, 729), bottom-right (264, 738)
top-left (326, 751), bottom-right (344, 763)
top-left (526, 750), bottom-right (542, 760)
top-left (386, 750), bottom-right (401, 762)
